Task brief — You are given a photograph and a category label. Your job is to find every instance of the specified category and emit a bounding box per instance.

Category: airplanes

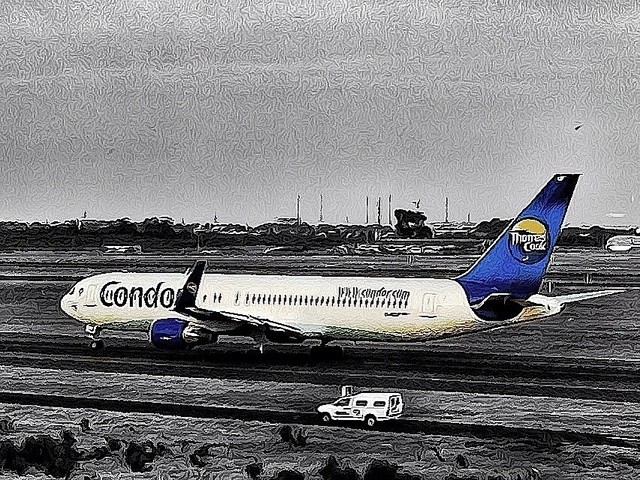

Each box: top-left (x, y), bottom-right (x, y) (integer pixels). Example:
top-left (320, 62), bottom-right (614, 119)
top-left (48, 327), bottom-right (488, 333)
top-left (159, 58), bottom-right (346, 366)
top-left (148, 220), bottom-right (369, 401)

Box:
top-left (57, 172), bottom-right (640, 367)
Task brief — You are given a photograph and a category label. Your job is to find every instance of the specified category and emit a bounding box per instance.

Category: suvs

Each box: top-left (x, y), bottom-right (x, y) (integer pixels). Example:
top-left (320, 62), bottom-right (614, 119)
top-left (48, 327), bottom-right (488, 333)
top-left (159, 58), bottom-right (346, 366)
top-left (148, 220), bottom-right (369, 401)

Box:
top-left (317, 386), bottom-right (402, 429)
